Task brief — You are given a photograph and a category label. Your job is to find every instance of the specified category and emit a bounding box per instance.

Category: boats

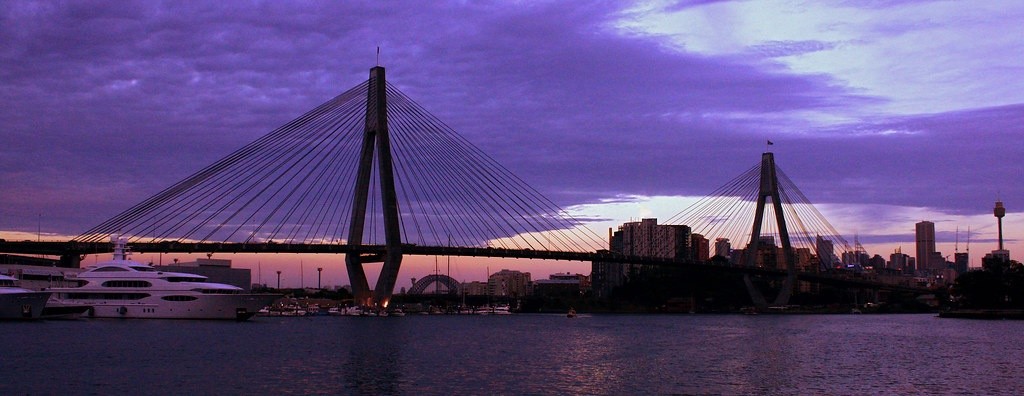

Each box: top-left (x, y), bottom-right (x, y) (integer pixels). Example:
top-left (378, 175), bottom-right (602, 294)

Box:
top-left (256, 300), bottom-right (512, 318)
top-left (0, 272), bottom-right (55, 321)
top-left (42, 230), bottom-right (285, 321)
top-left (567, 307), bottom-right (577, 317)
